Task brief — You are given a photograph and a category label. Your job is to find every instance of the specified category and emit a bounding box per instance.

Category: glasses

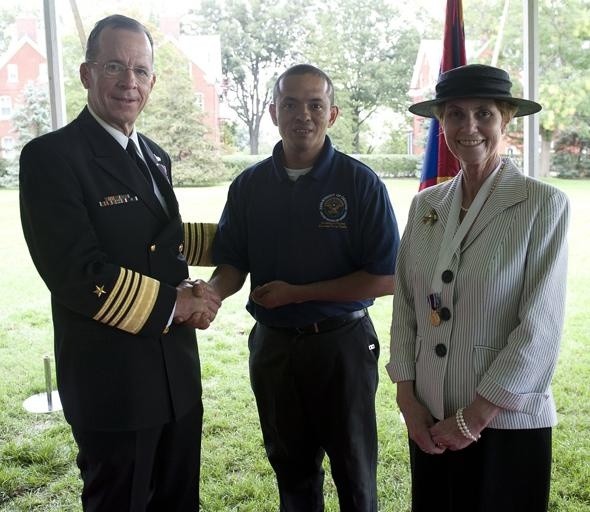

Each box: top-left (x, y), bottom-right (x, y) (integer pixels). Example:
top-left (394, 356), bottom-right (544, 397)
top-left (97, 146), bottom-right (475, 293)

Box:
top-left (93, 61), bottom-right (135, 76)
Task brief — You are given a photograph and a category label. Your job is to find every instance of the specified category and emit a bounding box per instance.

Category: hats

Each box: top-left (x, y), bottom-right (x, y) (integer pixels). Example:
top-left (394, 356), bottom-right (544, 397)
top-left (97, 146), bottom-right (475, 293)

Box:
top-left (408, 64), bottom-right (542, 118)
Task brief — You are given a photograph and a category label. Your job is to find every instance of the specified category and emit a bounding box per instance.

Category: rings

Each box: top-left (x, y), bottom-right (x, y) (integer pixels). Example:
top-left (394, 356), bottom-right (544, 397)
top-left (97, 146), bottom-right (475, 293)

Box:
top-left (435, 442), bottom-right (442, 448)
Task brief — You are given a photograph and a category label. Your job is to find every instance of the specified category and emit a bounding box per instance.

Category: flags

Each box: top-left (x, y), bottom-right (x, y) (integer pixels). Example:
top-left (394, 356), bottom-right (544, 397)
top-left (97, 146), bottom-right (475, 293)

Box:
top-left (417, 0), bottom-right (467, 192)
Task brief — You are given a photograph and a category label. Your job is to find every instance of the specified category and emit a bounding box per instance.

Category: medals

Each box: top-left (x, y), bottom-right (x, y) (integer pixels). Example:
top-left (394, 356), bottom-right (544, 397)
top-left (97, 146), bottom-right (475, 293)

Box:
top-left (430, 309), bottom-right (441, 327)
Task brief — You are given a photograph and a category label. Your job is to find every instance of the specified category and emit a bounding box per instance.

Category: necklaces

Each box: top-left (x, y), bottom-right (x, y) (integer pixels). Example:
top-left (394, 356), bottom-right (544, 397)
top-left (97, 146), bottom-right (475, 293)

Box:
top-left (461, 159), bottom-right (505, 213)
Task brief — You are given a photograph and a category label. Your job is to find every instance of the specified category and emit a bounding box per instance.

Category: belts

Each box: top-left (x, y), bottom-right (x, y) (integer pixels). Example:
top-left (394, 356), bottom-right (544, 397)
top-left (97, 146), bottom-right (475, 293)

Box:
top-left (303, 307), bottom-right (368, 336)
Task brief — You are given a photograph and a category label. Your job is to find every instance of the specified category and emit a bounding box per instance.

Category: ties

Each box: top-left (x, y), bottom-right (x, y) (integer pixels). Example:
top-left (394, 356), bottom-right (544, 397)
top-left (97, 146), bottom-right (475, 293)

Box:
top-left (126, 138), bottom-right (154, 193)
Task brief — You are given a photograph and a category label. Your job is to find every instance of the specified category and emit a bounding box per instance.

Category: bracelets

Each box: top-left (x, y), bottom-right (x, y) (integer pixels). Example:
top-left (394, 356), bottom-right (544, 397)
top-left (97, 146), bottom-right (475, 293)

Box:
top-left (455, 406), bottom-right (480, 442)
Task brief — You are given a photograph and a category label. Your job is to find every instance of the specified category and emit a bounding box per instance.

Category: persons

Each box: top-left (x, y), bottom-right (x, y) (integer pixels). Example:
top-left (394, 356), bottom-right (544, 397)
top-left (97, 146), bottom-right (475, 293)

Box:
top-left (386, 65), bottom-right (569, 511)
top-left (18, 13), bottom-right (221, 512)
top-left (174, 63), bottom-right (401, 512)
